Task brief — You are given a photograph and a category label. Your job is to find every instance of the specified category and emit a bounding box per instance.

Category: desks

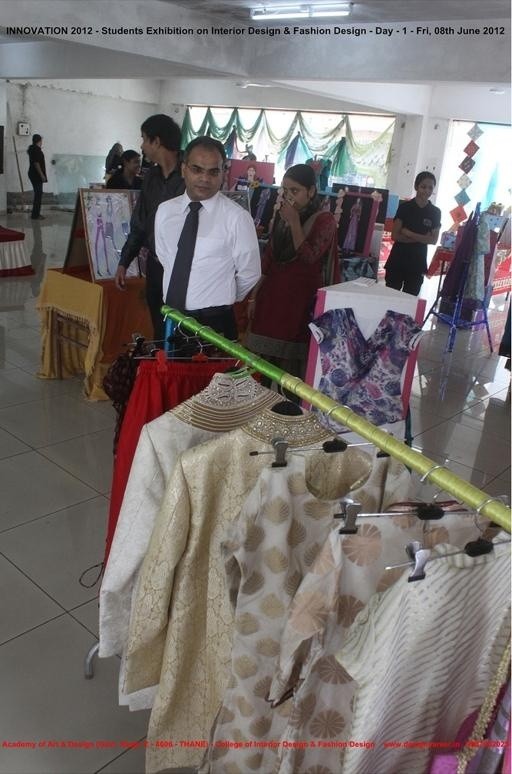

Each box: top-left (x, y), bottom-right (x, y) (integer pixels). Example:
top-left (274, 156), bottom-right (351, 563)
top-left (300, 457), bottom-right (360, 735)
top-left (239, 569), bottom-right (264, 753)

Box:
top-left (435, 242), bottom-right (512, 307)
top-left (33, 262), bottom-right (250, 406)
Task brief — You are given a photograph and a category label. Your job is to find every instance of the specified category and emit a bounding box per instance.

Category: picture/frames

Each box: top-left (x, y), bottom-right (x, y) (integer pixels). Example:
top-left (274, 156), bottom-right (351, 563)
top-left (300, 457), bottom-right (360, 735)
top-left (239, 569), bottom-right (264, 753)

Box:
top-left (60, 186), bottom-right (141, 286)
top-left (219, 188), bottom-right (251, 215)
top-left (61, 188), bottom-right (90, 275)
top-left (131, 189), bottom-right (151, 278)
top-left (236, 179), bottom-right (388, 260)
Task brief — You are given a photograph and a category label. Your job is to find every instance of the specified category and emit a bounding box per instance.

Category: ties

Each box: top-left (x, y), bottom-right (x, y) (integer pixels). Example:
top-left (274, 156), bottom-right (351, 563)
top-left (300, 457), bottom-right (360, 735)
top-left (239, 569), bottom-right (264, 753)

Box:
top-left (166, 202), bottom-right (202, 315)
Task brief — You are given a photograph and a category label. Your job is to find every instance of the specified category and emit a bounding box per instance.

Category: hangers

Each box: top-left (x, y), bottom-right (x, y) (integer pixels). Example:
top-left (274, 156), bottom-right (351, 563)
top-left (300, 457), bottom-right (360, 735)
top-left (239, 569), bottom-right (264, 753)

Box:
top-left (117, 303), bottom-right (511, 583)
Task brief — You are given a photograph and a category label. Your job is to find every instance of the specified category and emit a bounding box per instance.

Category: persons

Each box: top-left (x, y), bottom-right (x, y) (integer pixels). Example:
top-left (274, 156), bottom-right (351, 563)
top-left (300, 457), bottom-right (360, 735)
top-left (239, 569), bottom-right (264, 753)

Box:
top-left (107, 150), bottom-right (143, 189)
top-left (105, 144), bottom-right (123, 183)
top-left (241, 164), bottom-right (341, 382)
top-left (30, 221), bottom-right (47, 297)
top-left (154, 136), bottom-right (262, 341)
top-left (28, 134), bottom-right (47, 219)
top-left (383, 172), bottom-right (441, 296)
top-left (115, 114), bottom-right (186, 339)
top-left (235, 166), bottom-right (262, 191)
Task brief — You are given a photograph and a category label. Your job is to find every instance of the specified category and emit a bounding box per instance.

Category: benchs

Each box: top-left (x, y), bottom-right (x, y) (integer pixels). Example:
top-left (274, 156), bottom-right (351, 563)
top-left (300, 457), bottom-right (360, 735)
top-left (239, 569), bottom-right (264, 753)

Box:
top-left (1, 225), bottom-right (32, 271)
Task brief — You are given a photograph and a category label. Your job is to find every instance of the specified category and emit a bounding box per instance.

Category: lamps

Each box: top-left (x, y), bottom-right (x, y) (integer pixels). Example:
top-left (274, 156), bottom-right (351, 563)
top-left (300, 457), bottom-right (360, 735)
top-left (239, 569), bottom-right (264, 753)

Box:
top-left (248, 0), bottom-right (354, 20)
